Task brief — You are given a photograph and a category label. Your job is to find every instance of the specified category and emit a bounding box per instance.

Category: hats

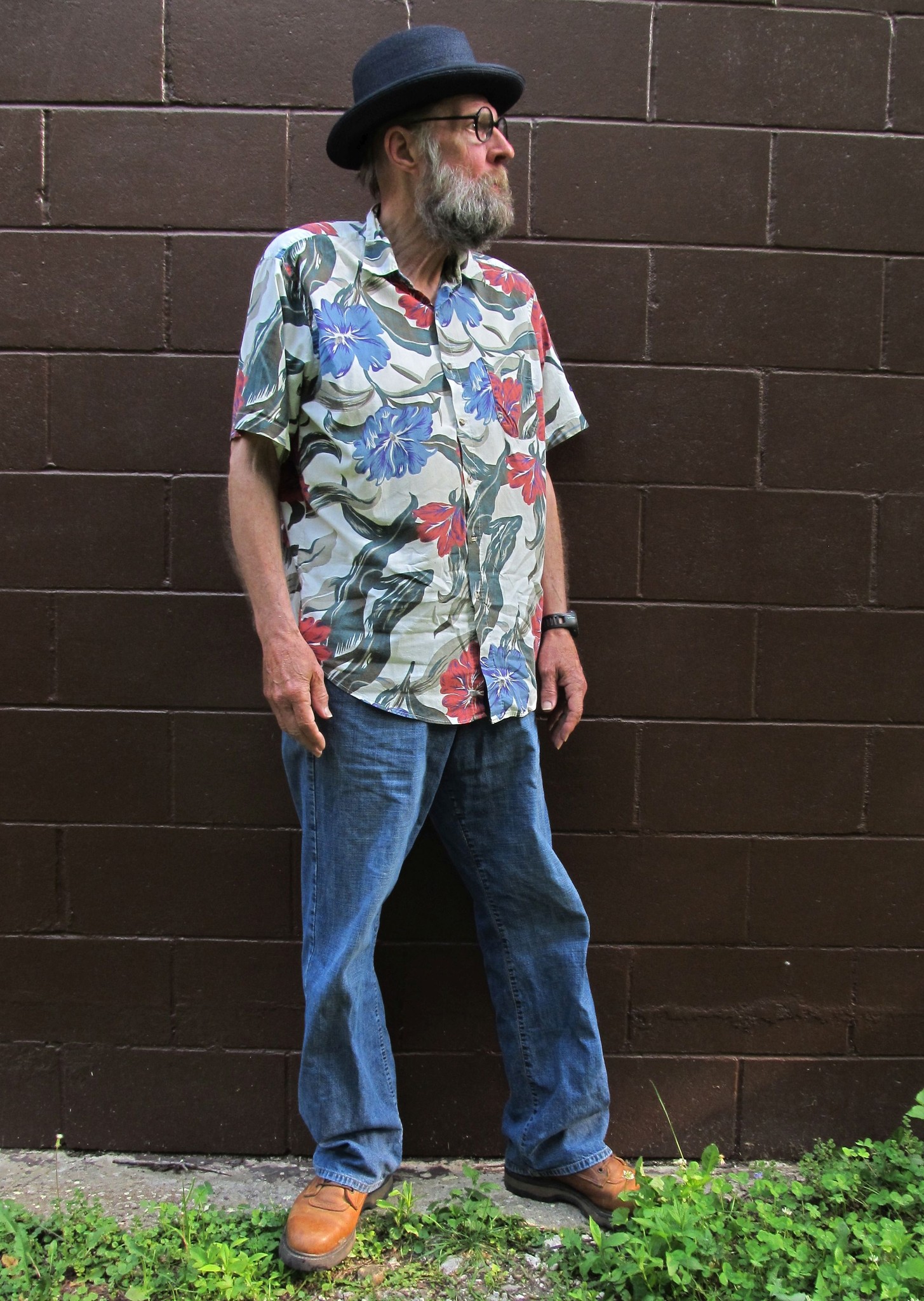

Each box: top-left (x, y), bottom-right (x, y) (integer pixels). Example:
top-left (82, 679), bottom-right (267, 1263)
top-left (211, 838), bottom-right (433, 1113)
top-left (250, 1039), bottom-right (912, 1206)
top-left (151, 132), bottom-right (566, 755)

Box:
top-left (327, 25), bottom-right (526, 170)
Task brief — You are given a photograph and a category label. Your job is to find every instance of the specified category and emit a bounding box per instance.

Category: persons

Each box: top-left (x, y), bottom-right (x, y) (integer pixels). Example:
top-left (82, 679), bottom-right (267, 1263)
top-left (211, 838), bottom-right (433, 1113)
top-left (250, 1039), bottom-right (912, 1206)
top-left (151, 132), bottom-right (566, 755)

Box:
top-left (230, 25), bottom-right (646, 1276)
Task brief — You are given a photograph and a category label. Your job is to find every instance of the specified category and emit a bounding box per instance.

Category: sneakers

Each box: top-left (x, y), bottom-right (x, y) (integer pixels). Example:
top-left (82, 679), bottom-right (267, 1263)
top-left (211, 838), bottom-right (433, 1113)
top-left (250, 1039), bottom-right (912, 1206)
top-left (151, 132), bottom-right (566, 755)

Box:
top-left (278, 1172), bottom-right (393, 1271)
top-left (505, 1154), bottom-right (637, 1226)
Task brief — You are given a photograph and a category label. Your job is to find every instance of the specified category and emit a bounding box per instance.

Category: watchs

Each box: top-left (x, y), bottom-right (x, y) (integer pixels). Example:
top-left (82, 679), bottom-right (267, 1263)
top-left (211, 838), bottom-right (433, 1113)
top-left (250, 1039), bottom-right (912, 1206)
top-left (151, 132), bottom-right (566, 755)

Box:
top-left (545, 605), bottom-right (580, 639)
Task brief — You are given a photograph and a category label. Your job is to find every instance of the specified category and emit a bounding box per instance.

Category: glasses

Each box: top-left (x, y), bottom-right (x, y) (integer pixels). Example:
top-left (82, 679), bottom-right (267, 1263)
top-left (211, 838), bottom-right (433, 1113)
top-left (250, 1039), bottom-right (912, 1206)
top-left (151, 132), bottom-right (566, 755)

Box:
top-left (403, 106), bottom-right (508, 142)
